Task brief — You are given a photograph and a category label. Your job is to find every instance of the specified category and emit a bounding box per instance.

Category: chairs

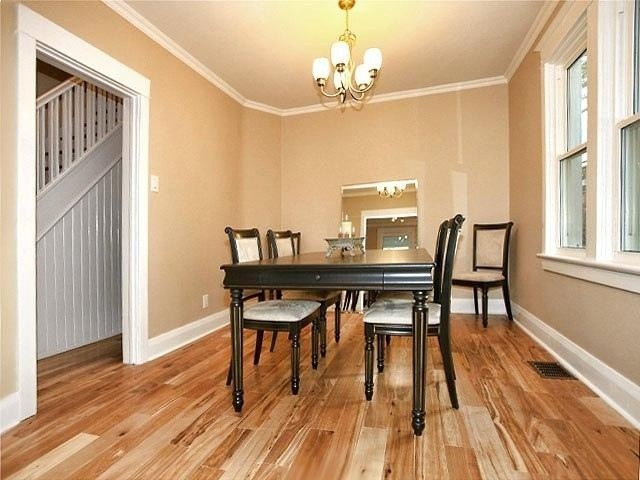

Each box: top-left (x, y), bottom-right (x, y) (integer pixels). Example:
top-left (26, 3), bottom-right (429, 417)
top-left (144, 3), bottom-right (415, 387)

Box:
top-left (225, 224), bottom-right (346, 394)
top-left (360, 215), bottom-right (466, 410)
top-left (452, 222), bottom-right (514, 329)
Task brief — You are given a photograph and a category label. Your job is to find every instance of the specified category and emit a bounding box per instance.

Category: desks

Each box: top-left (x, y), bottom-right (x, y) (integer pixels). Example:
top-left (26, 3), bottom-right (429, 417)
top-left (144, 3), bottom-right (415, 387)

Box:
top-left (218, 248), bottom-right (438, 437)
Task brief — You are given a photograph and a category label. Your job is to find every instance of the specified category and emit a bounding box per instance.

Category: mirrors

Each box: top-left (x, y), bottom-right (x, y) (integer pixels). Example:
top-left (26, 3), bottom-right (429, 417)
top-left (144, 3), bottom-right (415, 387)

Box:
top-left (340, 177), bottom-right (420, 300)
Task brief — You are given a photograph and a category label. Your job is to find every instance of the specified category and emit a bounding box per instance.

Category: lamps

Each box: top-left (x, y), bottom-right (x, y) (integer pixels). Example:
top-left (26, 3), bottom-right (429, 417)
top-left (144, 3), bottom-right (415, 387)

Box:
top-left (312, 1), bottom-right (383, 104)
top-left (376, 182), bottom-right (406, 202)
